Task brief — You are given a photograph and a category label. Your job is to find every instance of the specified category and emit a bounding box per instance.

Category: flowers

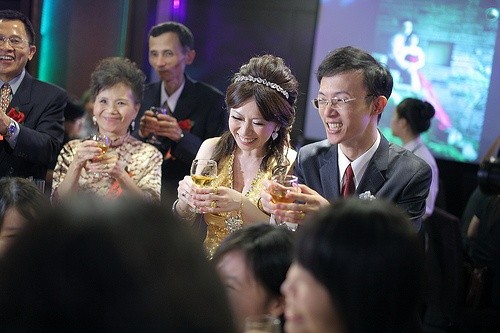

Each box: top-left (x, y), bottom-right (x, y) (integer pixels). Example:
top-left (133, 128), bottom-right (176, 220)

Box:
top-left (7, 106), bottom-right (25, 127)
top-left (178, 119), bottom-right (194, 132)
top-left (358, 190), bottom-right (376, 201)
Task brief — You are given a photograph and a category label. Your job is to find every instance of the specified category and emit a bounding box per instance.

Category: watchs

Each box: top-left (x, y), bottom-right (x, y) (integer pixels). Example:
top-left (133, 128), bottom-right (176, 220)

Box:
top-left (1, 118), bottom-right (15, 138)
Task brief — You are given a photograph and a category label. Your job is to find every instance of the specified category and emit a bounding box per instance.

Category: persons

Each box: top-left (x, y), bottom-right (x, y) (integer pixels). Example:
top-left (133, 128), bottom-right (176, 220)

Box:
top-left (64, 94), bottom-right (85, 142)
top-left (0, 10), bottom-right (65, 179)
top-left (261, 45), bottom-right (432, 234)
top-left (129, 21), bottom-right (228, 215)
top-left (172, 53), bottom-right (300, 261)
top-left (0, 176), bottom-right (438, 333)
top-left (50, 57), bottom-right (163, 210)
top-left (391, 98), bottom-right (438, 257)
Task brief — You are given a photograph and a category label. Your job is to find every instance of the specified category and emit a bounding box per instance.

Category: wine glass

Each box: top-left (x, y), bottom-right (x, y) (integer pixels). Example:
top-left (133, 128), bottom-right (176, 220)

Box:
top-left (271, 174), bottom-right (299, 232)
top-left (146, 107), bottom-right (166, 145)
top-left (188, 159), bottom-right (219, 214)
top-left (89, 133), bottom-right (110, 179)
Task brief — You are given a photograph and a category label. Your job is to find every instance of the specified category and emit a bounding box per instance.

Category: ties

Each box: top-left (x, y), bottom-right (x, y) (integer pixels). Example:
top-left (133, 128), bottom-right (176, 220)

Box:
top-left (0, 84), bottom-right (12, 115)
top-left (161, 100), bottom-right (173, 116)
top-left (341, 164), bottom-right (356, 199)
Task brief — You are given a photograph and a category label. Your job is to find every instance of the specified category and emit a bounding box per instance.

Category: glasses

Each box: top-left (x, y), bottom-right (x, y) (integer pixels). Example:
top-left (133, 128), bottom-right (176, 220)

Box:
top-left (312, 94), bottom-right (377, 109)
top-left (0, 35), bottom-right (33, 49)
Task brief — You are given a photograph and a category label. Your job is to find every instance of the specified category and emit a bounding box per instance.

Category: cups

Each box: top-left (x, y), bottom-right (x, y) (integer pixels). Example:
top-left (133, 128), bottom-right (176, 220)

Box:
top-left (243, 314), bottom-right (282, 333)
